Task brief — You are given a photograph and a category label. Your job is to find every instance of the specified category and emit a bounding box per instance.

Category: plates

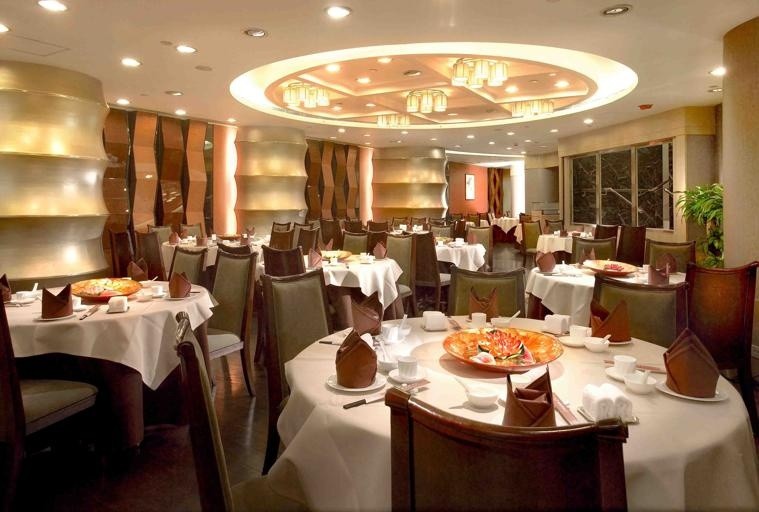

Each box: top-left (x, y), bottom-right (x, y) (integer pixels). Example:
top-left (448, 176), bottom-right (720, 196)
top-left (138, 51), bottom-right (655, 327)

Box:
top-left (560, 335), bottom-right (592, 348)
top-left (656, 379), bottom-right (729, 402)
top-left (389, 366), bottom-right (426, 383)
top-left (537, 269), bottom-right (560, 275)
top-left (443, 326), bottom-right (565, 374)
top-left (5, 289), bottom-right (199, 322)
top-left (319, 250), bottom-right (351, 261)
top-left (70, 277), bottom-right (142, 301)
top-left (583, 259), bottom-right (640, 277)
top-left (326, 371), bottom-right (387, 392)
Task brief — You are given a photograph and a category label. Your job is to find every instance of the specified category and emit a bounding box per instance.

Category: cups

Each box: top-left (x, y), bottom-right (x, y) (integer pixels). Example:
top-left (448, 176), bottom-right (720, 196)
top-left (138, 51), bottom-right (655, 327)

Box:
top-left (397, 356), bottom-right (418, 376)
top-left (381, 324), bottom-right (399, 341)
top-left (471, 313), bottom-right (487, 328)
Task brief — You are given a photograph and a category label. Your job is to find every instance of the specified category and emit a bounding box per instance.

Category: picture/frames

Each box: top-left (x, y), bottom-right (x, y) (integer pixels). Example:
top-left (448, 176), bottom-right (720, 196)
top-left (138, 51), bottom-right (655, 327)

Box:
top-left (464, 173), bottom-right (476, 202)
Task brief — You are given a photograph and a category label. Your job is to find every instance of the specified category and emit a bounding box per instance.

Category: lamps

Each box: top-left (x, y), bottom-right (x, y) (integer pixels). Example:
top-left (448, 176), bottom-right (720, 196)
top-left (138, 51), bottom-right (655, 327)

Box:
top-left (280, 78), bottom-right (330, 114)
top-left (449, 56), bottom-right (511, 90)
top-left (511, 96), bottom-right (554, 119)
top-left (398, 87), bottom-right (448, 115)
top-left (374, 115), bottom-right (415, 130)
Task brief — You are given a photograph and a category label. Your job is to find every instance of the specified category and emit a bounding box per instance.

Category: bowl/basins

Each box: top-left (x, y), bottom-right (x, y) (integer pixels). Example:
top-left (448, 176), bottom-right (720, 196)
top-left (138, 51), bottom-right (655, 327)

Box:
top-left (490, 318), bottom-right (514, 327)
top-left (568, 325), bottom-right (657, 395)
top-left (450, 315), bottom-right (470, 327)
top-left (376, 348), bottom-right (405, 370)
top-left (396, 324), bottom-right (412, 337)
top-left (466, 386), bottom-right (501, 407)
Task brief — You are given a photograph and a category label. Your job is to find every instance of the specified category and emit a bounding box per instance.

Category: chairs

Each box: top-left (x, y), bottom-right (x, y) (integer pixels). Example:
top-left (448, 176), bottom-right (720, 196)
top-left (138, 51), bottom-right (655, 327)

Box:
top-left (0, 209), bottom-right (759, 510)
